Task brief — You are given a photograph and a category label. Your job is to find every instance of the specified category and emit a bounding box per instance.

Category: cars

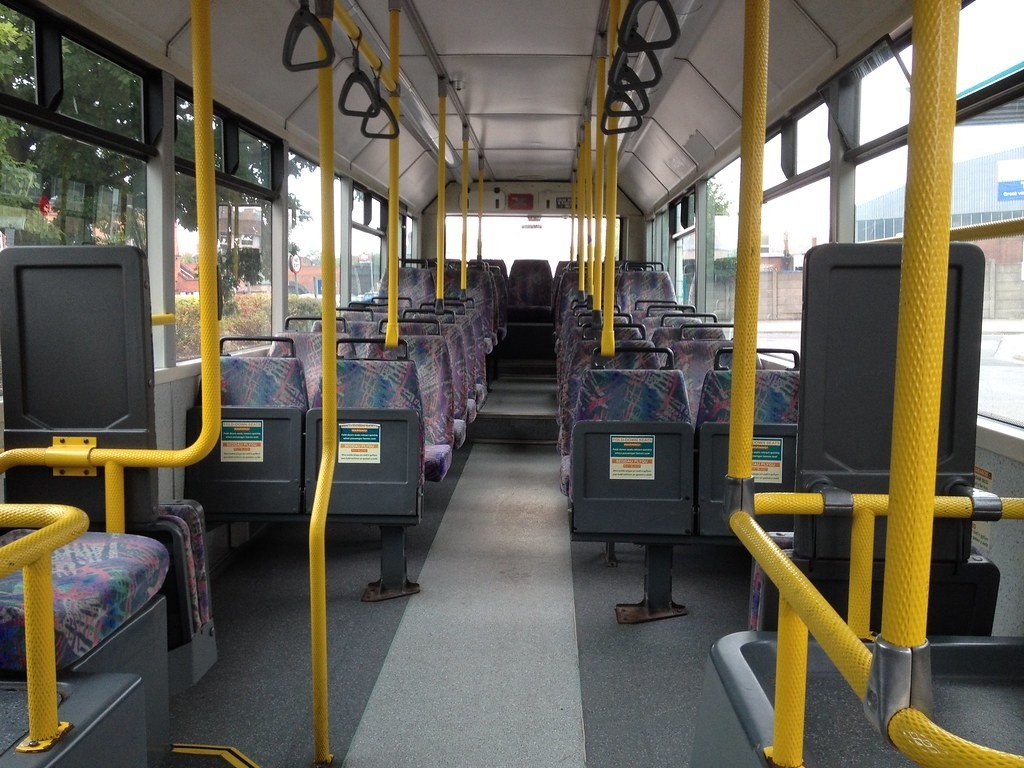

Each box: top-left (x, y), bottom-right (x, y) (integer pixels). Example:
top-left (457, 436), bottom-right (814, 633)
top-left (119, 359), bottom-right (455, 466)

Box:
top-left (288, 282), bottom-right (310, 296)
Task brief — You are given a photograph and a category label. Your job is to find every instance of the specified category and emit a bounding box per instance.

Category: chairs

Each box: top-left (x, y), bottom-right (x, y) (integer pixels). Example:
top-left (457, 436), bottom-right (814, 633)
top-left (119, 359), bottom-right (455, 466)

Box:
top-left (0, 243), bottom-right (218, 696)
top-left (748, 242), bottom-right (999, 638)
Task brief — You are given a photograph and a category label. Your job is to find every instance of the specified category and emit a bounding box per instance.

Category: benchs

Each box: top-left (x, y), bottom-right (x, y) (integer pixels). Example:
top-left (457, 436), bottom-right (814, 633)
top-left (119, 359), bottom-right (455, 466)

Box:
top-left (187, 259), bottom-right (799, 623)
